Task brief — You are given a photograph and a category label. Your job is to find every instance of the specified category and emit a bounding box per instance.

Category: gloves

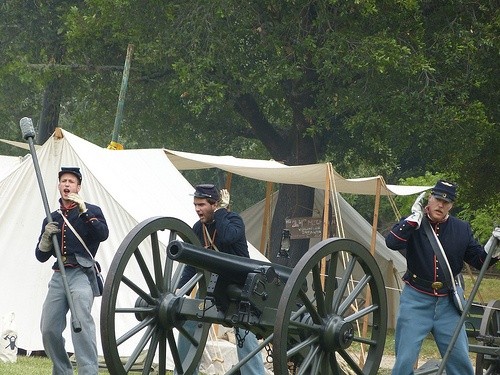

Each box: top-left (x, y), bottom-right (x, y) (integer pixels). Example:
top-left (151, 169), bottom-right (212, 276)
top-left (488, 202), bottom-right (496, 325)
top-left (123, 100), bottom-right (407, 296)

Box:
top-left (67, 193), bottom-right (87, 214)
top-left (219, 190), bottom-right (230, 208)
top-left (38, 222), bottom-right (61, 252)
top-left (404, 192), bottom-right (427, 229)
top-left (484, 227), bottom-right (500, 258)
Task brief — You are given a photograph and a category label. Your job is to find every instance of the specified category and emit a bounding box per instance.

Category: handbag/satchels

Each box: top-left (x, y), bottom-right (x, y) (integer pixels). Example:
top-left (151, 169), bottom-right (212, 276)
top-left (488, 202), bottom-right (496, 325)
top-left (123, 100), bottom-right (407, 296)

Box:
top-left (74, 253), bottom-right (104, 297)
top-left (450, 273), bottom-right (467, 313)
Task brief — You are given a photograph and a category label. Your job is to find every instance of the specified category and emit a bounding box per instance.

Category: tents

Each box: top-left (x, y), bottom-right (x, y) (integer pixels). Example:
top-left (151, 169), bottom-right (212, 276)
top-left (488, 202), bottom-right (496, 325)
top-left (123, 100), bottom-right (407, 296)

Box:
top-left (0, 126), bottom-right (434, 368)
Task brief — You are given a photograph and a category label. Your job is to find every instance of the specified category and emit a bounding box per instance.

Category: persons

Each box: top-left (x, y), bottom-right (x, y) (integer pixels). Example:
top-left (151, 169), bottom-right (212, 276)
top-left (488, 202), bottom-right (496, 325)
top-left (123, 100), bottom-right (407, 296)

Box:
top-left (386, 180), bottom-right (500, 375)
top-left (35, 167), bottom-right (109, 375)
top-left (174, 184), bottom-right (268, 375)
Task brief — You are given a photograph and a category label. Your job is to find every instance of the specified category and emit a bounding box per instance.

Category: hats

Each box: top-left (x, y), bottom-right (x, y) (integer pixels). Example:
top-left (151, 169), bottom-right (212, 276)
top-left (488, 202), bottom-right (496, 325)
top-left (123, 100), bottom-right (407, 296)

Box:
top-left (432, 180), bottom-right (458, 203)
top-left (59, 167), bottom-right (82, 180)
top-left (189, 184), bottom-right (221, 202)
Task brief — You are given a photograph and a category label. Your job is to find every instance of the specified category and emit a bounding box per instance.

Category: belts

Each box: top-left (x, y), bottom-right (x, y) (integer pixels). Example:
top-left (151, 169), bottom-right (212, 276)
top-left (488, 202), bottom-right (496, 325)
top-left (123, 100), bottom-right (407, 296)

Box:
top-left (60, 256), bottom-right (77, 264)
top-left (407, 269), bottom-right (447, 290)
